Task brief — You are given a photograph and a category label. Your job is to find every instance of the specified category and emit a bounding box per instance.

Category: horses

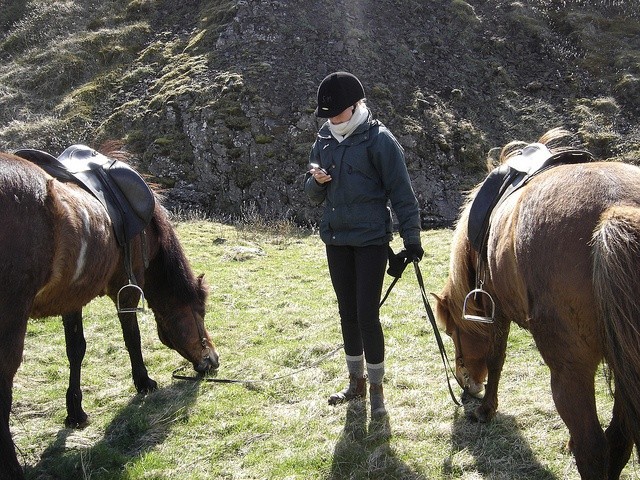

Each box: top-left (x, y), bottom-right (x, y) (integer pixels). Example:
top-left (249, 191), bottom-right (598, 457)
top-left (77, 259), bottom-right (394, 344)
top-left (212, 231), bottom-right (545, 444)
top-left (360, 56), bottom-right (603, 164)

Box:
top-left (0, 140), bottom-right (219, 480)
top-left (429, 129), bottom-right (640, 480)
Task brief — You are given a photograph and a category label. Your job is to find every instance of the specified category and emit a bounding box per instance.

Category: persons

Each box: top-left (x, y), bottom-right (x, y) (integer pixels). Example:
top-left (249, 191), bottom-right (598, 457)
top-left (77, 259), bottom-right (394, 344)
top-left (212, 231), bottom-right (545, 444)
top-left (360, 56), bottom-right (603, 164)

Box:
top-left (303, 71), bottom-right (425, 417)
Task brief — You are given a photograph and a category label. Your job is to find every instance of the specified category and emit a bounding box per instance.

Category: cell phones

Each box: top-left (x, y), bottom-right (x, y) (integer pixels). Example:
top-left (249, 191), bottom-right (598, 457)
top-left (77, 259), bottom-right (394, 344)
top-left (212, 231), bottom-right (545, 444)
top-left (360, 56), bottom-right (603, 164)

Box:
top-left (310, 163), bottom-right (327, 176)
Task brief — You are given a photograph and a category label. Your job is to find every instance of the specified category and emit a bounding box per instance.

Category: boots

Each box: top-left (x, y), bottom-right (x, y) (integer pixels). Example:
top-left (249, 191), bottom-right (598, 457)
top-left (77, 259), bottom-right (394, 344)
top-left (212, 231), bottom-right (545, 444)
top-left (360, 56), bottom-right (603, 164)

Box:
top-left (370, 383), bottom-right (387, 417)
top-left (328, 375), bottom-right (366, 404)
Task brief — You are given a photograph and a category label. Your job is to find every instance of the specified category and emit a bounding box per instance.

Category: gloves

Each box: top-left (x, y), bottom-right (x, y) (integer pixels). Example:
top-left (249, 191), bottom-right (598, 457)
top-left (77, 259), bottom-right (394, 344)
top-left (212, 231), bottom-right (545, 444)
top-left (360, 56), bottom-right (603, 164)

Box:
top-left (386, 244), bottom-right (411, 278)
top-left (405, 244), bottom-right (424, 263)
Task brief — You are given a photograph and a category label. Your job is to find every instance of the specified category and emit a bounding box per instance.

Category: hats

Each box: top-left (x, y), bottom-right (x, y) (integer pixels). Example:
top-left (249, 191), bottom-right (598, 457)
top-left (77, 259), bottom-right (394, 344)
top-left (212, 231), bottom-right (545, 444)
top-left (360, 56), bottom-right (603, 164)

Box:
top-left (314, 72), bottom-right (365, 118)
